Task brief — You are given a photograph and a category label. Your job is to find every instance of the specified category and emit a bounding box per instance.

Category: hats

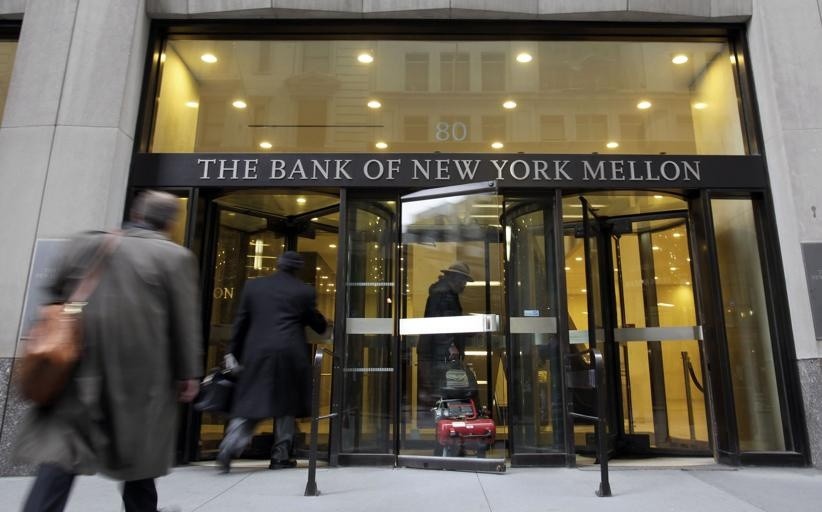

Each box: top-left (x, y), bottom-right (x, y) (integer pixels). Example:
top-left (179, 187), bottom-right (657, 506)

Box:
top-left (439, 261), bottom-right (475, 283)
top-left (274, 248), bottom-right (306, 273)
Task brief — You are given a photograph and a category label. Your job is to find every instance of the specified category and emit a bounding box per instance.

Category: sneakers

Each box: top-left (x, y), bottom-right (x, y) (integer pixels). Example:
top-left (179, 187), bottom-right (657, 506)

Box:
top-left (215, 449), bottom-right (233, 472)
top-left (266, 455), bottom-right (298, 470)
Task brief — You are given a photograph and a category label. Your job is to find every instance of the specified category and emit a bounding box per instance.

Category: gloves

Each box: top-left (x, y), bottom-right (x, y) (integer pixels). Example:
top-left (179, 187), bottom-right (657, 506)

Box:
top-left (224, 353), bottom-right (244, 374)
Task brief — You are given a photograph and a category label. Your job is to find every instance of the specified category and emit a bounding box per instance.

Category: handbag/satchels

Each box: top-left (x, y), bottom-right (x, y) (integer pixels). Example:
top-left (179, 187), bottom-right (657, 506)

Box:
top-left (193, 364), bottom-right (240, 415)
top-left (13, 297), bottom-right (85, 409)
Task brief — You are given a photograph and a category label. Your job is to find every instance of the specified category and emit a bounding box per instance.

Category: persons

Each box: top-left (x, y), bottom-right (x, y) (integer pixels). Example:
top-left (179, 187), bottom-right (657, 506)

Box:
top-left (15, 188), bottom-right (202, 512)
top-left (214, 249), bottom-right (329, 472)
top-left (413, 258), bottom-right (476, 420)
